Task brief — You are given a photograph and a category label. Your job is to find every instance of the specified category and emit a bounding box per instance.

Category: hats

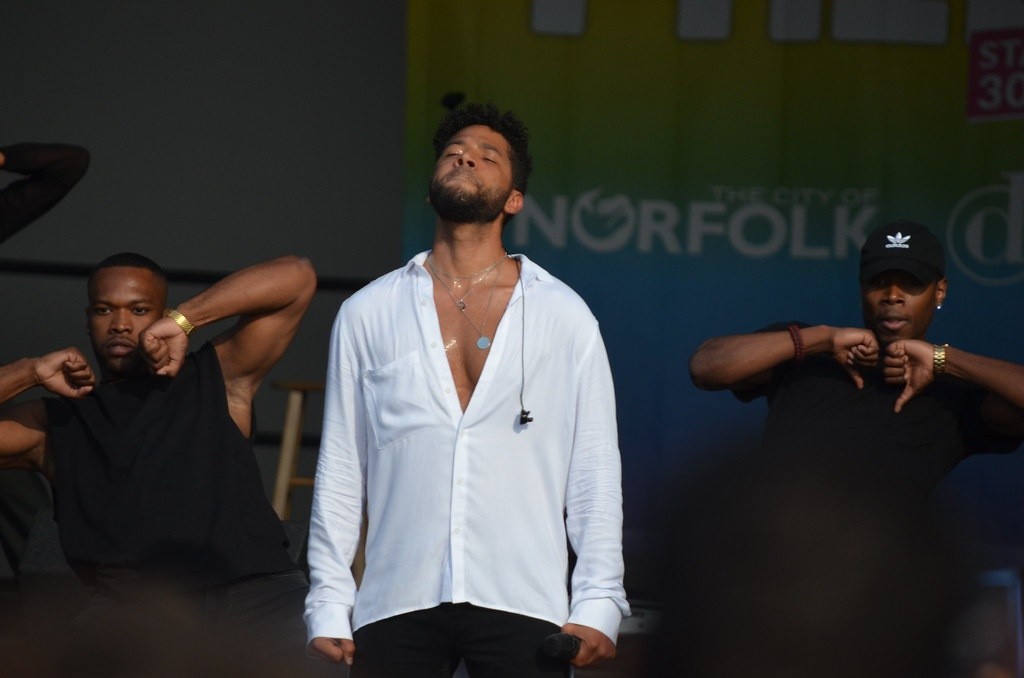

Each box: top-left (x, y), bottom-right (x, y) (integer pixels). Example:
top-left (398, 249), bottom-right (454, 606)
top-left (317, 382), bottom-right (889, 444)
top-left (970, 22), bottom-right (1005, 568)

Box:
top-left (859, 224), bottom-right (945, 288)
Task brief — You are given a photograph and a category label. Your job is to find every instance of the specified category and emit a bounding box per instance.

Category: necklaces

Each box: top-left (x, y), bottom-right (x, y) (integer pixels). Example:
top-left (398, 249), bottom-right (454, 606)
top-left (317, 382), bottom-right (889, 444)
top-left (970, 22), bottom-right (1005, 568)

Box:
top-left (426, 251), bottom-right (508, 348)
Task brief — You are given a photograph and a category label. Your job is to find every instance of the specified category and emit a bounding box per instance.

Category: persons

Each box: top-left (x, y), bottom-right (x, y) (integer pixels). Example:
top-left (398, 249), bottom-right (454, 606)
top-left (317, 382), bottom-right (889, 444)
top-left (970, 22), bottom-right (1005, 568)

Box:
top-left (302, 102), bottom-right (633, 677)
top-left (688, 219), bottom-right (1023, 678)
top-left (0, 251), bottom-right (352, 678)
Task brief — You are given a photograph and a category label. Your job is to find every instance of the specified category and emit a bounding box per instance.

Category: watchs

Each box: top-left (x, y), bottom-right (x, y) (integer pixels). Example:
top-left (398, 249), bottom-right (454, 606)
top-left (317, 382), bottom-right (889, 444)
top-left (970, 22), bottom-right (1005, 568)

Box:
top-left (161, 305), bottom-right (194, 337)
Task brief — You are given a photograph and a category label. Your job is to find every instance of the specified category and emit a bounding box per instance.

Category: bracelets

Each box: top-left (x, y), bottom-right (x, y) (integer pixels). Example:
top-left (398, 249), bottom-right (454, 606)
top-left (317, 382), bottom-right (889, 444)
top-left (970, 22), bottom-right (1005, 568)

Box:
top-left (788, 323), bottom-right (805, 360)
top-left (934, 344), bottom-right (946, 375)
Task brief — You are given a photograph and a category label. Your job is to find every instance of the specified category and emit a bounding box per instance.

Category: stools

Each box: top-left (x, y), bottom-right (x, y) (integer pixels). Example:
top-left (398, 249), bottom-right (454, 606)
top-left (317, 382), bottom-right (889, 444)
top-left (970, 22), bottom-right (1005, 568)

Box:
top-left (269, 382), bottom-right (326, 523)
